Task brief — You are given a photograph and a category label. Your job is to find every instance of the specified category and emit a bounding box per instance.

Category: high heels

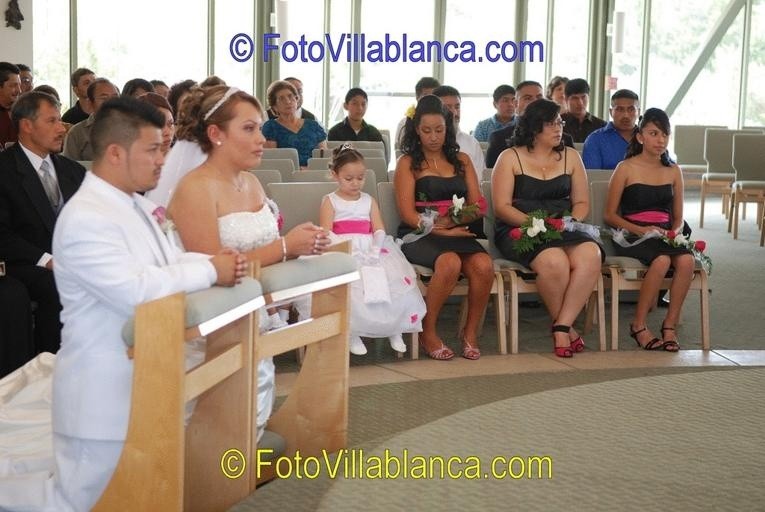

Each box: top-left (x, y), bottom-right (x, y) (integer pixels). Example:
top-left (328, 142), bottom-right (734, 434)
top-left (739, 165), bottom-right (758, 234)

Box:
top-left (629, 324), bottom-right (665, 350)
top-left (570, 336), bottom-right (586, 352)
top-left (551, 320), bottom-right (574, 358)
top-left (662, 320), bottom-right (680, 352)
top-left (419, 334), bottom-right (455, 360)
top-left (462, 335), bottom-right (481, 360)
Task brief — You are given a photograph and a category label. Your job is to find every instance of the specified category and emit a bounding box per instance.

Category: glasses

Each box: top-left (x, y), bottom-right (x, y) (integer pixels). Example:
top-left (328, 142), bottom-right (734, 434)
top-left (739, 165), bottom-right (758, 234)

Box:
top-left (278, 95), bottom-right (300, 103)
top-left (544, 121), bottom-right (566, 127)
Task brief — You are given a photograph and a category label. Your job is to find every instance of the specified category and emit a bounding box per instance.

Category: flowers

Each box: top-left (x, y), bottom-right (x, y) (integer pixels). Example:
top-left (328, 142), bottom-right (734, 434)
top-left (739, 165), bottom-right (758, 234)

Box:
top-left (613, 227), bottom-right (712, 276)
top-left (508, 207), bottom-right (604, 254)
top-left (412, 191), bottom-right (489, 235)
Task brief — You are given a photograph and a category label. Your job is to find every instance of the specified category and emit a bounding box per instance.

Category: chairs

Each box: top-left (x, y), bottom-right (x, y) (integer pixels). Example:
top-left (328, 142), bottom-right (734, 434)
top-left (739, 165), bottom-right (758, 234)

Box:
top-left (480, 180), bottom-right (608, 355)
top-left (728, 134), bottom-right (765, 240)
top-left (266, 181), bottom-right (405, 363)
top-left (591, 179), bottom-right (712, 351)
top-left (674, 126), bottom-right (728, 220)
top-left (699, 126), bottom-right (765, 248)
top-left (377, 182), bottom-right (508, 360)
top-left (237, 129), bottom-right (615, 191)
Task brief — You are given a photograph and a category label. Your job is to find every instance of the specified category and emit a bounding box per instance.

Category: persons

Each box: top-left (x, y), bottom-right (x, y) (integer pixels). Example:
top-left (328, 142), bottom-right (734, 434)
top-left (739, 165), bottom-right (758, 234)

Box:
top-left (319, 144), bottom-right (424, 355)
top-left (257, 81), bottom-right (324, 166)
top-left (0, 59), bottom-right (254, 159)
top-left (395, 79), bottom-right (441, 147)
top-left (1, 98), bottom-right (247, 510)
top-left (3, 76), bottom-right (331, 470)
top-left (5, 91), bottom-right (84, 378)
top-left (602, 108), bottom-right (695, 352)
top-left (473, 84), bottom-right (521, 138)
top-left (582, 89), bottom-right (693, 307)
top-left (491, 102), bottom-right (605, 359)
top-left (395, 88), bottom-right (483, 185)
top-left (555, 80), bottom-right (608, 142)
top-left (486, 82), bottom-right (577, 308)
top-left (325, 89), bottom-right (384, 141)
top-left (546, 76), bottom-right (571, 113)
top-left (389, 96), bottom-right (491, 360)
top-left (261, 77), bottom-right (316, 121)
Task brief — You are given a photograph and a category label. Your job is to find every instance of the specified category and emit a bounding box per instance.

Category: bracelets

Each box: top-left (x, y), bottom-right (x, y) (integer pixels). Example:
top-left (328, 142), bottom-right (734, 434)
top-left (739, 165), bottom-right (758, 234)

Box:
top-left (280, 236), bottom-right (288, 262)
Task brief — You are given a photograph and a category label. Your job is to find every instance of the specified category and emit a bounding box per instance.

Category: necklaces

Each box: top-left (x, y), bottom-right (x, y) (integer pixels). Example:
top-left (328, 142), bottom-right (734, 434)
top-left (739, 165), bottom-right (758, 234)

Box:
top-left (205, 161), bottom-right (248, 195)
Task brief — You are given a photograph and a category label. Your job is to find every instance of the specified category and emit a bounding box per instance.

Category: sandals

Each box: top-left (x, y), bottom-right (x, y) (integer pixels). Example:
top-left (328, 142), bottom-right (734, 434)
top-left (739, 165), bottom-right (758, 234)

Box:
top-left (390, 335), bottom-right (406, 353)
top-left (350, 336), bottom-right (367, 355)
